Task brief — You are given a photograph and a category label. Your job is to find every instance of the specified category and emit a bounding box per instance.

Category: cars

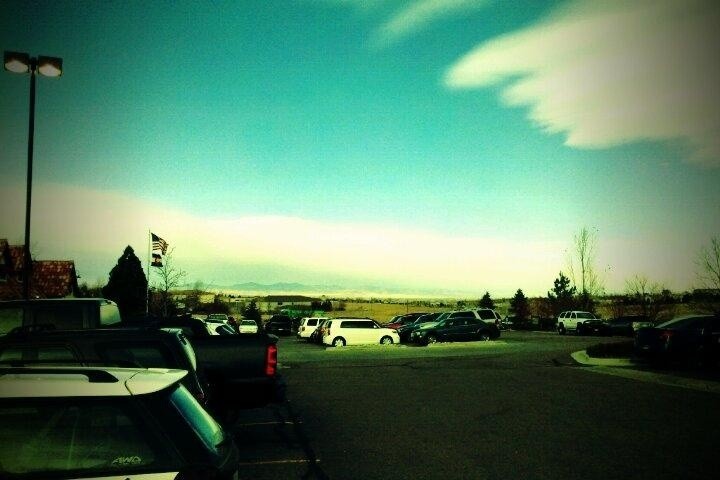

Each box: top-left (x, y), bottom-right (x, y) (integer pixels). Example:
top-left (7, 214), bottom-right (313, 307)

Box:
top-left (607, 312), bottom-right (718, 329)
top-left (634, 316), bottom-right (720, 372)
top-left (293, 308), bottom-right (503, 346)
top-left (194, 311), bottom-right (295, 345)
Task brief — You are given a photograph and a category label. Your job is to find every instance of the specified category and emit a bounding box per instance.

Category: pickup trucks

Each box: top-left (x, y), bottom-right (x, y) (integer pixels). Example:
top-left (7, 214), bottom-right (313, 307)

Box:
top-left (0, 296), bottom-right (286, 430)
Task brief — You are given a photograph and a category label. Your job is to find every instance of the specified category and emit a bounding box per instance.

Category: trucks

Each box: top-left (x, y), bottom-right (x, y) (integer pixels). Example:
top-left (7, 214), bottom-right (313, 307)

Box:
top-left (557, 310), bottom-right (605, 334)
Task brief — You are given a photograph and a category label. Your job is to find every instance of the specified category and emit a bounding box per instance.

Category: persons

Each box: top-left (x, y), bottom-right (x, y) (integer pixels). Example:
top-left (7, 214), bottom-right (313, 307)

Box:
top-left (227, 317), bottom-right (239, 334)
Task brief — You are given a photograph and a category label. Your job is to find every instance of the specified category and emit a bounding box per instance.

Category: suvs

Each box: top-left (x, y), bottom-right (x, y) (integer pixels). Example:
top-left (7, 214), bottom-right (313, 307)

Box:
top-left (1, 323), bottom-right (210, 411)
top-left (0, 367), bottom-right (243, 480)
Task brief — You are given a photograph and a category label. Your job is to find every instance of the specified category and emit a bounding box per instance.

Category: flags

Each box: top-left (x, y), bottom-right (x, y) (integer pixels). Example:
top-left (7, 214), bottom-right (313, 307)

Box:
top-left (151, 232), bottom-right (168, 255)
top-left (151, 253), bottom-right (163, 267)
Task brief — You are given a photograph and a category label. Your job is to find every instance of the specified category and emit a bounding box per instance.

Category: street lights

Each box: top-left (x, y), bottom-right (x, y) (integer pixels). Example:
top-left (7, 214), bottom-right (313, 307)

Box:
top-left (2, 49), bottom-right (64, 298)
